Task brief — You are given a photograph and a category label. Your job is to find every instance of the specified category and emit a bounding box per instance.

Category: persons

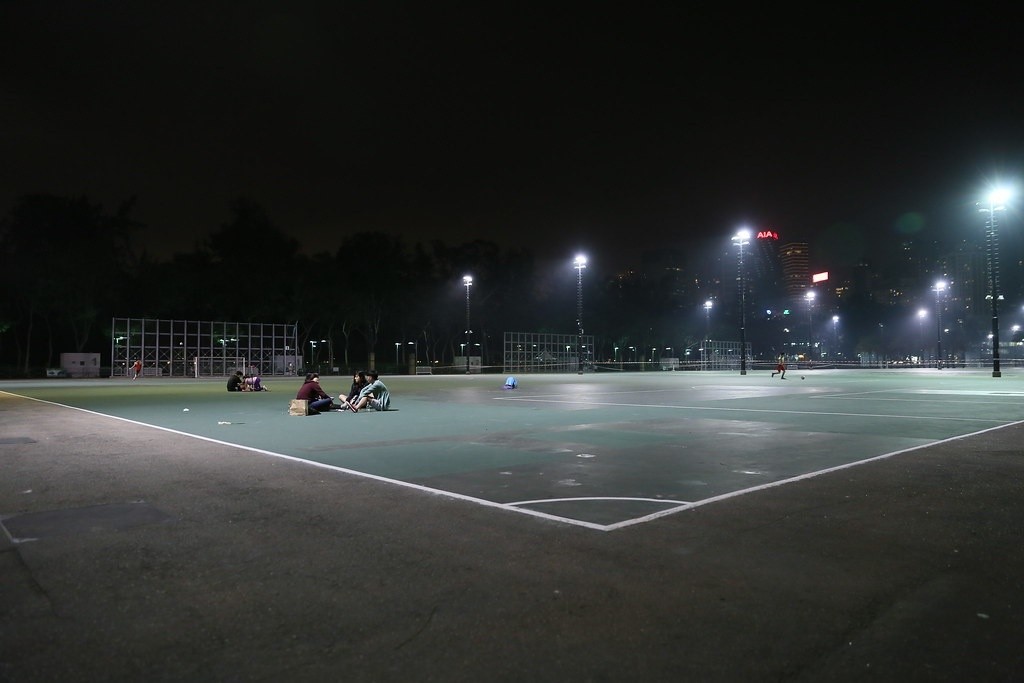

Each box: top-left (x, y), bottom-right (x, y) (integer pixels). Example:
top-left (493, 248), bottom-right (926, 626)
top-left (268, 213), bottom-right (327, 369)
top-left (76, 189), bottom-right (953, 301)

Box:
top-left (227, 371), bottom-right (243, 392)
top-left (346, 370), bottom-right (390, 413)
top-left (128, 357), bottom-right (142, 380)
top-left (338, 369), bottom-right (370, 410)
top-left (295, 374), bottom-right (334, 413)
top-left (241, 376), bottom-right (268, 391)
top-left (504, 376), bottom-right (517, 389)
top-left (772, 352), bottom-right (786, 379)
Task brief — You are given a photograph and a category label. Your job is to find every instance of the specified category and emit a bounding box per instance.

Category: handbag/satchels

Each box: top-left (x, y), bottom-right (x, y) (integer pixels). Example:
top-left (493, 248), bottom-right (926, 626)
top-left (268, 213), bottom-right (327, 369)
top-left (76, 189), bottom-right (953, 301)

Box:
top-left (289, 399), bottom-right (308, 416)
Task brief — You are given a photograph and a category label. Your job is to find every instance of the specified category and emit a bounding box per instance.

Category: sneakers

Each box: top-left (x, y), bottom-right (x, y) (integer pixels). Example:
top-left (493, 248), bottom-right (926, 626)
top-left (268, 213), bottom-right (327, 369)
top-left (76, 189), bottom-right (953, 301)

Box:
top-left (346, 401), bottom-right (358, 413)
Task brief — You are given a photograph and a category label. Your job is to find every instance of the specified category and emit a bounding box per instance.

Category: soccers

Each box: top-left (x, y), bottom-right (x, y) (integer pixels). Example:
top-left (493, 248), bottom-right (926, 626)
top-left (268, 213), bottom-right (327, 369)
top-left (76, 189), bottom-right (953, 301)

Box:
top-left (801, 375), bottom-right (805, 379)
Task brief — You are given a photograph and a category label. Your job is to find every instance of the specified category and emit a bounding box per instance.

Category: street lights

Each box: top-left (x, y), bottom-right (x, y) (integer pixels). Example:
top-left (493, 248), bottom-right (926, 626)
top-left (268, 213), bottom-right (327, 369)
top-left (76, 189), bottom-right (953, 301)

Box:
top-left (573, 256), bottom-right (588, 374)
top-left (975, 189), bottom-right (1007, 378)
top-left (930, 282), bottom-right (946, 369)
top-left (919, 310), bottom-right (926, 368)
top-left (462, 275), bottom-right (473, 374)
top-left (731, 230), bottom-right (752, 374)
top-left (803, 291), bottom-right (817, 370)
top-left (704, 301), bottom-right (713, 370)
top-left (832, 314), bottom-right (841, 369)
